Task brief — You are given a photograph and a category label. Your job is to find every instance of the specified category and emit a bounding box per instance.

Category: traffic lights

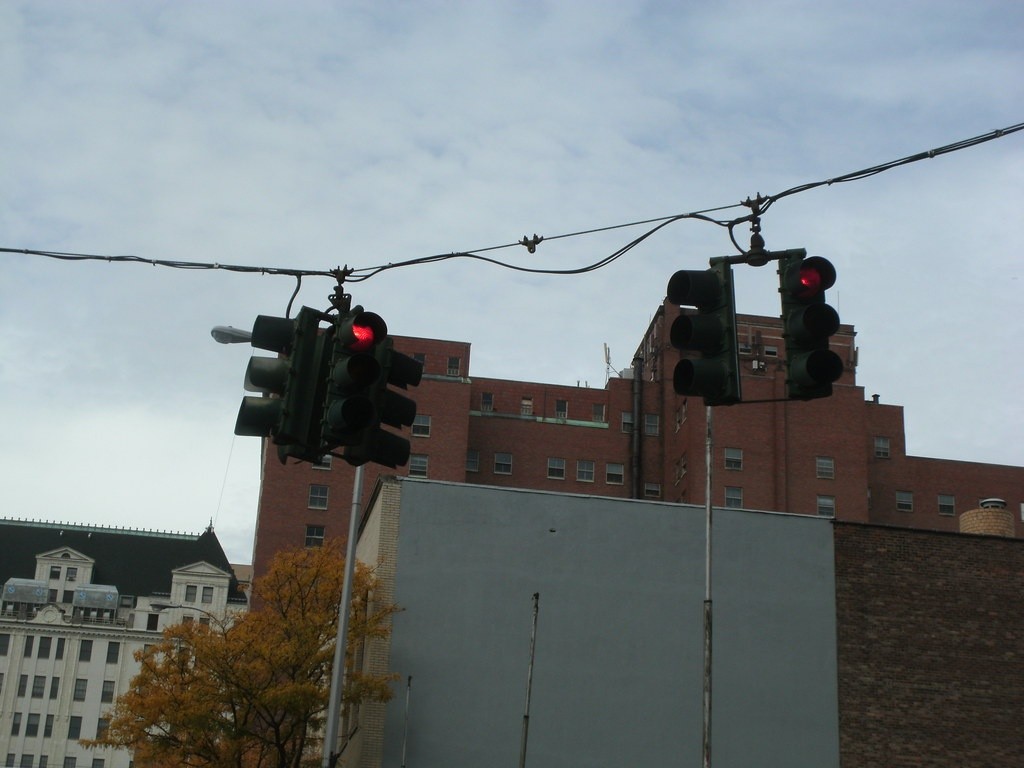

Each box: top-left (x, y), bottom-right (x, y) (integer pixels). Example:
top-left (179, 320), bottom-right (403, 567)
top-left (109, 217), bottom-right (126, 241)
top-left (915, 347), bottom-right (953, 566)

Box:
top-left (346, 330), bottom-right (423, 472)
top-left (319, 305), bottom-right (390, 444)
top-left (668, 257), bottom-right (744, 408)
top-left (776, 257), bottom-right (845, 401)
top-left (234, 306), bottom-right (329, 450)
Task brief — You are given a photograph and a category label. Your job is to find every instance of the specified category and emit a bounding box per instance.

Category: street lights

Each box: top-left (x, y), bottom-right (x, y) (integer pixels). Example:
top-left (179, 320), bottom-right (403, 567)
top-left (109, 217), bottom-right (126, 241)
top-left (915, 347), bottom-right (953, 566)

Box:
top-left (208, 323), bottom-right (367, 768)
